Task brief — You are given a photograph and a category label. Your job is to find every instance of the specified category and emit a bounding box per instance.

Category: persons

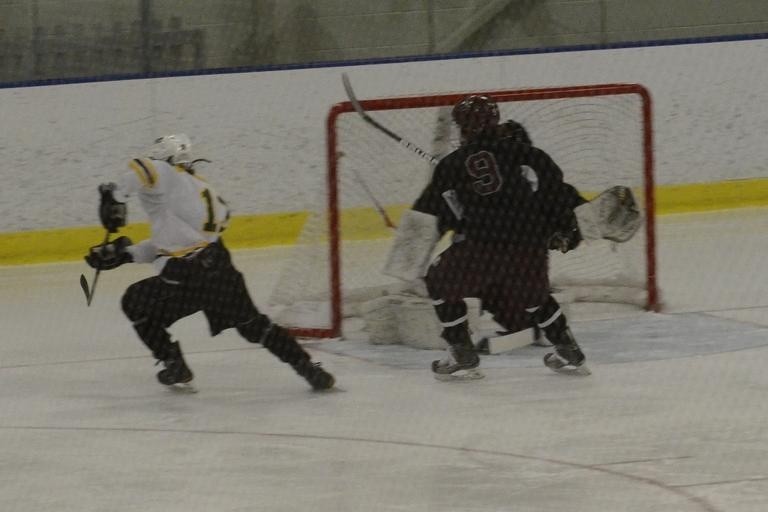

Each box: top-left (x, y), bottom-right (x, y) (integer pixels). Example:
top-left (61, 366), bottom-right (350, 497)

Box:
top-left (84, 131), bottom-right (335, 395)
top-left (409, 94), bottom-right (586, 376)
top-left (476, 120), bottom-right (591, 354)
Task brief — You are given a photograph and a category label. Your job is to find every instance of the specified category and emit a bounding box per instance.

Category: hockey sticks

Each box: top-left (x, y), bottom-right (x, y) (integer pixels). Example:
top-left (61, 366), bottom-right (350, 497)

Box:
top-left (80, 230), bottom-right (111, 305)
top-left (469, 321), bottom-right (540, 355)
top-left (342, 74), bottom-right (439, 166)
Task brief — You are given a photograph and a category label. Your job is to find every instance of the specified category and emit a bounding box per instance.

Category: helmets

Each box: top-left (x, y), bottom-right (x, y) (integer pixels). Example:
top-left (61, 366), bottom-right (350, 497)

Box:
top-left (451, 95), bottom-right (499, 135)
top-left (148, 133), bottom-right (194, 167)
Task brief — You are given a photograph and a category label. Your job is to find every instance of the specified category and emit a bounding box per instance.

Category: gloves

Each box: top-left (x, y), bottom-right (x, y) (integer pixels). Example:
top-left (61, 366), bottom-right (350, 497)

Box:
top-left (99, 180), bottom-right (127, 235)
top-left (84, 237), bottom-right (134, 270)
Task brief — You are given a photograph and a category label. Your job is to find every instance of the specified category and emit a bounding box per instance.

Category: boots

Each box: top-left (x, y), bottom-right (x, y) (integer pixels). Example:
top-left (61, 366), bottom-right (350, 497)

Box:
top-left (545, 327), bottom-right (583, 370)
top-left (432, 334), bottom-right (481, 374)
top-left (296, 355), bottom-right (335, 391)
top-left (157, 341), bottom-right (192, 386)
